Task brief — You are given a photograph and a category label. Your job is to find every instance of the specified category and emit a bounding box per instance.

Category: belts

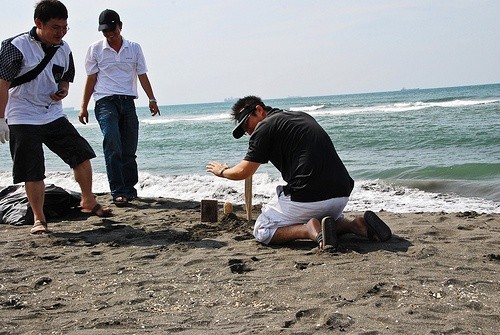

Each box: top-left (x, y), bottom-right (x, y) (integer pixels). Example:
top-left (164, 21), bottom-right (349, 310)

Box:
top-left (105, 94), bottom-right (137, 100)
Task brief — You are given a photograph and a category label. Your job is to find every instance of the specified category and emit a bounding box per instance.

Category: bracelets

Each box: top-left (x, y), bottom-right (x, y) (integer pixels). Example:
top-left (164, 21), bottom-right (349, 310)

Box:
top-left (220, 167), bottom-right (228, 176)
top-left (149, 99), bottom-right (156, 102)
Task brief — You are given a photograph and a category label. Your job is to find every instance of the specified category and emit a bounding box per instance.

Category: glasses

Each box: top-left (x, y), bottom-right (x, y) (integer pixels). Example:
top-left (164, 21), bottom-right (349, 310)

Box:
top-left (102, 24), bottom-right (117, 34)
top-left (48, 25), bottom-right (70, 34)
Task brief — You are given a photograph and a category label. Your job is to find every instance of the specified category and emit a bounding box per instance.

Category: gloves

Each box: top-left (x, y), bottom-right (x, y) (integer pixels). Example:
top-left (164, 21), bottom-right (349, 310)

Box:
top-left (0, 117), bottom-right (10, 144)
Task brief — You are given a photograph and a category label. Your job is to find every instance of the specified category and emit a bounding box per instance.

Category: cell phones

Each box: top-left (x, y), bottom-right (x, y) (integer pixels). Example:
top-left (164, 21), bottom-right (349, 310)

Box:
top-left (55, 89), bottom-right (64, 95)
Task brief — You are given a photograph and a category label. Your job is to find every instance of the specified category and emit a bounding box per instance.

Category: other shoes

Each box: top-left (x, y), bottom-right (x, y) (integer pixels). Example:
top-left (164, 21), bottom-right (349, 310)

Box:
top-left (113, 194), bottom-right (128, 208)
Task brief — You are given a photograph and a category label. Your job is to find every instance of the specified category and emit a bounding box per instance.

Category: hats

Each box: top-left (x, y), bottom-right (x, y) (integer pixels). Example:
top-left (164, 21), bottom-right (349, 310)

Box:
top-left (98, 9), bottom-right (120, 31)
top-left (232, 101), bottom-right (266, 140)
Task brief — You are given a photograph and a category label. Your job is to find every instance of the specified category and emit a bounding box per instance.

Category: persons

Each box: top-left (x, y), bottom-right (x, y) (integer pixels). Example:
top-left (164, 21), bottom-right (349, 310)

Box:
top-left (205, 96), bottom-right (392, 252)
top-left (0, 0), bottom-right (113, 237)
top-left (78, 9), bottom-right (161, 204)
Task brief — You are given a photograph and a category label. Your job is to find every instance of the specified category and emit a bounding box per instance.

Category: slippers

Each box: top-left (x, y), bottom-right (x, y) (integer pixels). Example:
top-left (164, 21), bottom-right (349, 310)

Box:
top-left (313, 216), bottom-right (336, 252)
top-left (30, 220), bottom-right (48, 237)
top-left (363, 210), bottom-right (392, 242)
top-left (80, 203), bottom-right (114, 217)
